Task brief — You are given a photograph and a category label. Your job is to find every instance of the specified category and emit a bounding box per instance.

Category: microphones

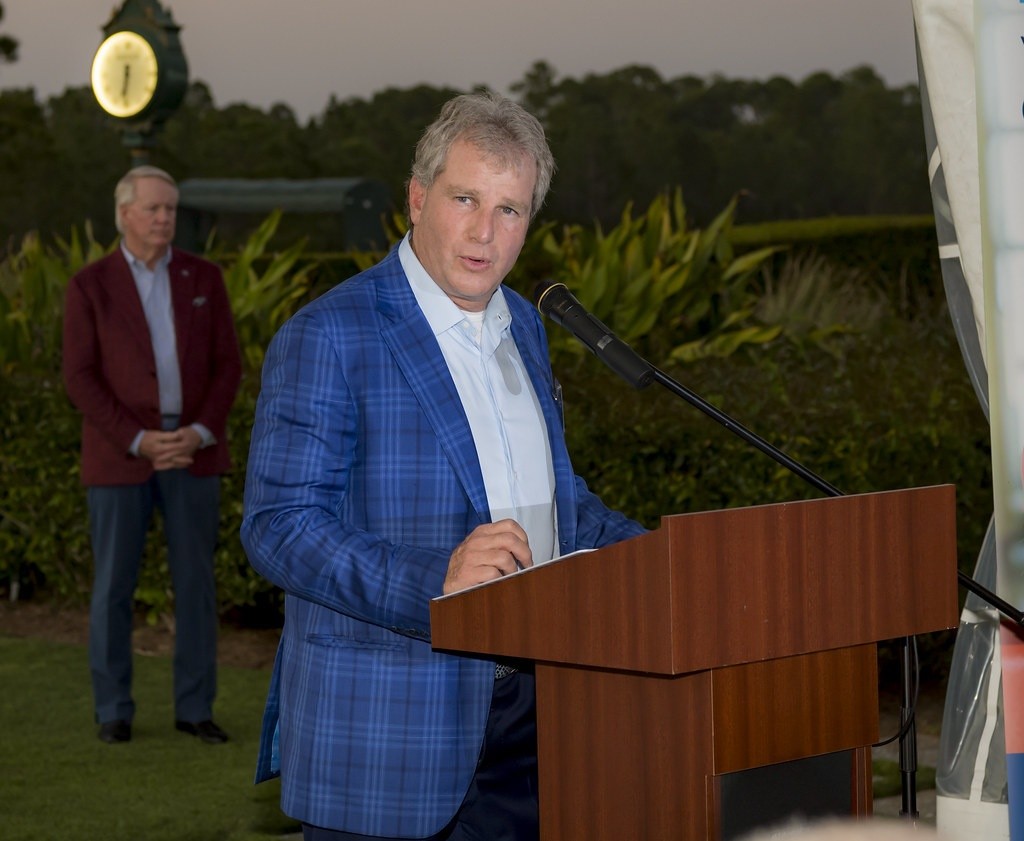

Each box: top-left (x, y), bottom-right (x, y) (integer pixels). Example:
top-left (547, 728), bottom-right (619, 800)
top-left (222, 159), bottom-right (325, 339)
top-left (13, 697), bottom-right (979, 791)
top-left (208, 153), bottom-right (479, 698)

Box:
top-left (532, 280), bottom-right (655, 390)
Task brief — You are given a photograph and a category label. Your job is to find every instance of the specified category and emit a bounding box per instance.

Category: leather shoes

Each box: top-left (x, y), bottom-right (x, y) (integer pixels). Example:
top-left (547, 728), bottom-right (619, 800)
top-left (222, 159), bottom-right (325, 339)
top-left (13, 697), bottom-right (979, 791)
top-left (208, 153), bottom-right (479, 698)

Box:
top-left (97, 718), bottom-right (132, 744)
top-left (174, 718), bottom-right (228, 745)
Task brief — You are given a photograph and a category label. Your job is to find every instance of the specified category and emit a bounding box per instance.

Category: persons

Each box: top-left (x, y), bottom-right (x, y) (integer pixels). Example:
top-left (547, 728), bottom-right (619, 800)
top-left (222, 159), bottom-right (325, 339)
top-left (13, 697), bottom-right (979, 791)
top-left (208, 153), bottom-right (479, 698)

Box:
top-left (239, 95), bottom-right (656, 839)
top-left (61, 165), bottom-right (243, 745)
